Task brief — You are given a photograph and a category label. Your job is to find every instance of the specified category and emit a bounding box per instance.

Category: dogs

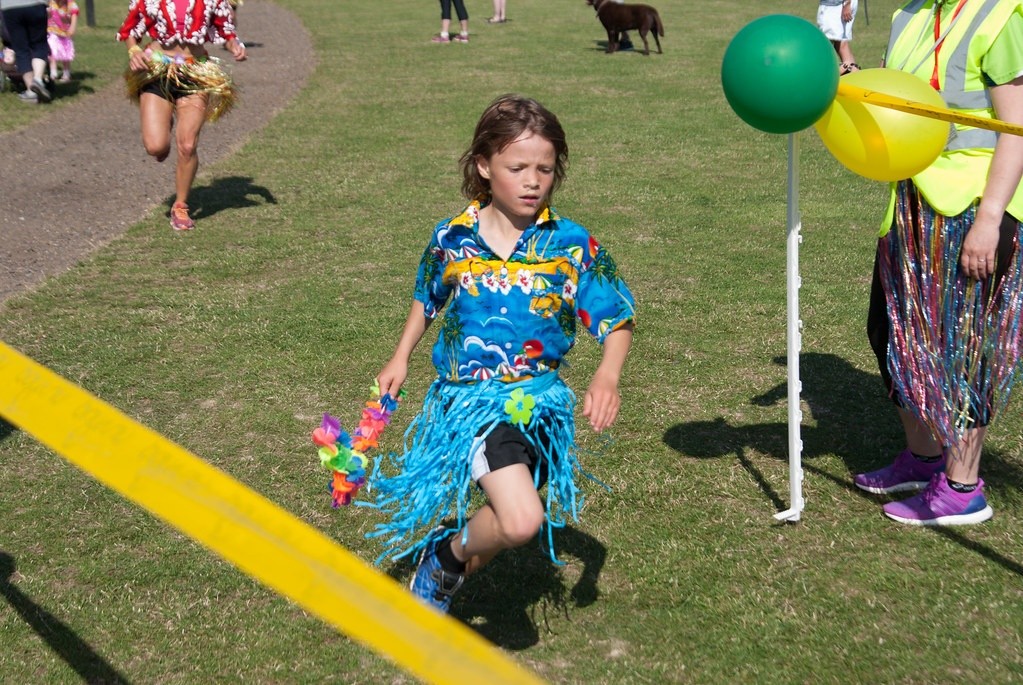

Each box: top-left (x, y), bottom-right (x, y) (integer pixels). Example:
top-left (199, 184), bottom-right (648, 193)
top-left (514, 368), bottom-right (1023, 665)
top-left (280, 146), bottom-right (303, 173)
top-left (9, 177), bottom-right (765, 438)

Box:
top-left (586, 0), bottom-right (665, 55)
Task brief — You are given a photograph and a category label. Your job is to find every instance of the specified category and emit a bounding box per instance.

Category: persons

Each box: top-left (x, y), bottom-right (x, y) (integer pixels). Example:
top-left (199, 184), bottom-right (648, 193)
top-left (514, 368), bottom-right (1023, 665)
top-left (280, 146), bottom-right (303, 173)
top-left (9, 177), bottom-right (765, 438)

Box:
top-left (0, 0), bottom-right (78, 103)
top-left (817, 0), bottom-right (862, 76)
top-left (373, 95), bottom-right (639, 614)
top-left (432, 0), bottom-right (470, 43)
top-left (853, 0), bottom-right (1023, 529)
top-left (487, 0), bottom-right (507, 24)
top-left (117, 0), bottom-right (246, 231)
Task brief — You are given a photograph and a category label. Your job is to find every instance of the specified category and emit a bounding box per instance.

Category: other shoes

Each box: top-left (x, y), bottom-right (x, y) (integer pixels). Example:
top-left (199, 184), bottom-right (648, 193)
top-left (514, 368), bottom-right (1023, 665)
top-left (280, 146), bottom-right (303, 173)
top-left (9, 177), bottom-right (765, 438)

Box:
top-left (46, 74), bottom-right (58, 81)
top-left (58, 74), bottom-right (70, 81)
top-left (488, 17), bottom-right (506, 23)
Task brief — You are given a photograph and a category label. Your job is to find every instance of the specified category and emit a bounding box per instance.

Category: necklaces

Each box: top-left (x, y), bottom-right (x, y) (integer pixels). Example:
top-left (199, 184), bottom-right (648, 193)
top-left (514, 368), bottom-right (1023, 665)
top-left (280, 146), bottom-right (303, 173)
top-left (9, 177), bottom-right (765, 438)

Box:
top-left (896, 0), bottom-right (966, 74)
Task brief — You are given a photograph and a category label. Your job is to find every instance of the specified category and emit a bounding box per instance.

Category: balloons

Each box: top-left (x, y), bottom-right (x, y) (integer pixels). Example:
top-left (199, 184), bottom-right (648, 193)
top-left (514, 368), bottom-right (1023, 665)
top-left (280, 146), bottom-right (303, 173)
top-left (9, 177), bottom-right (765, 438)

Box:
top-left (810, 68), bottom-right (950, 182)
top-left (720, 14), bottom-right (839, 135)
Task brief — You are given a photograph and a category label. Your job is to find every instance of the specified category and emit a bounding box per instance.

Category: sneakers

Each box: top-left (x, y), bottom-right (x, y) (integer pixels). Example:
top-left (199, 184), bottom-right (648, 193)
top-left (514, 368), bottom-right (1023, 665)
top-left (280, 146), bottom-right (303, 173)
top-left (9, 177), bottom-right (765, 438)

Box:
top-left (29, 78), bottom-right (53, 101)
top-left (454, 33), bottom-right (468, 41)
top-left (410, 524), bottom-right (466, 614)
top-left (169, 204), bottom-right (194, 231)
top-left (431, 35), bottom-right (451, 43)
top-left (19, 89), bottom-right (38, 102)
top-left (882, 472), bottom-right (993, 525)
top-left (854, 448), bottom-right (948, 495)
